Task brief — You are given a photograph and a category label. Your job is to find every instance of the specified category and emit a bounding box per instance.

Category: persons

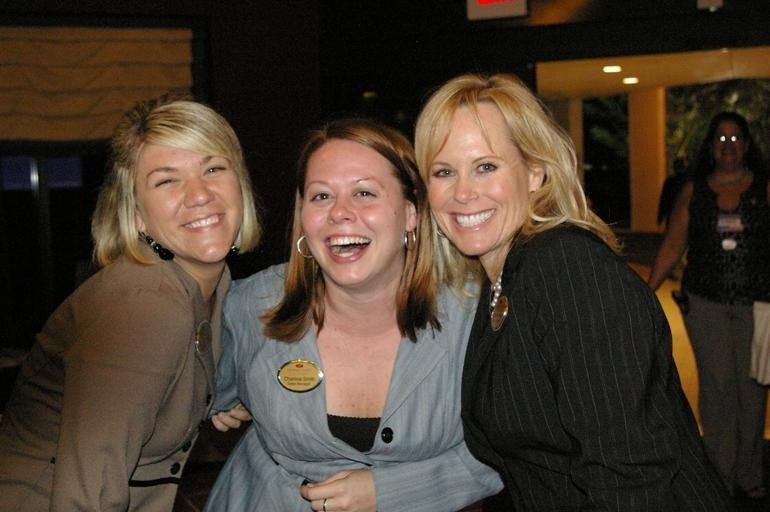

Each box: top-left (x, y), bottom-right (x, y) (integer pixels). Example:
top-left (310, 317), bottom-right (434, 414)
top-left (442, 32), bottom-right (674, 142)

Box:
top-left (655, 156), bottom-right (693, 269)
top-left (413, 73), bottom-right (736, 510)
top-left (1, 93), bottom-right (265, 512)
top-left (644, 111), bottom-right (769, 511)
top-left (206, 117), bottom-right (505, 511)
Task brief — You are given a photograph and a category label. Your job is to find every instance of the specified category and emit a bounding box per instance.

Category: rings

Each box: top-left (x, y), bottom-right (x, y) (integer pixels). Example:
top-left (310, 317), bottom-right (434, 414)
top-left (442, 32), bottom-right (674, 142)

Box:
top-left (321, 495), bottom-right (328, 510)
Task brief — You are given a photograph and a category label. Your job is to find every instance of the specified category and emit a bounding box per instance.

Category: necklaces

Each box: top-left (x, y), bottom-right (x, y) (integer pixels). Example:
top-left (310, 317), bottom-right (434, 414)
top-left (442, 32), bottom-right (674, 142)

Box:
top-left (486, 267), bottom-right (502, 309)
top-left (710, 170), bottom-right (748, 185)
